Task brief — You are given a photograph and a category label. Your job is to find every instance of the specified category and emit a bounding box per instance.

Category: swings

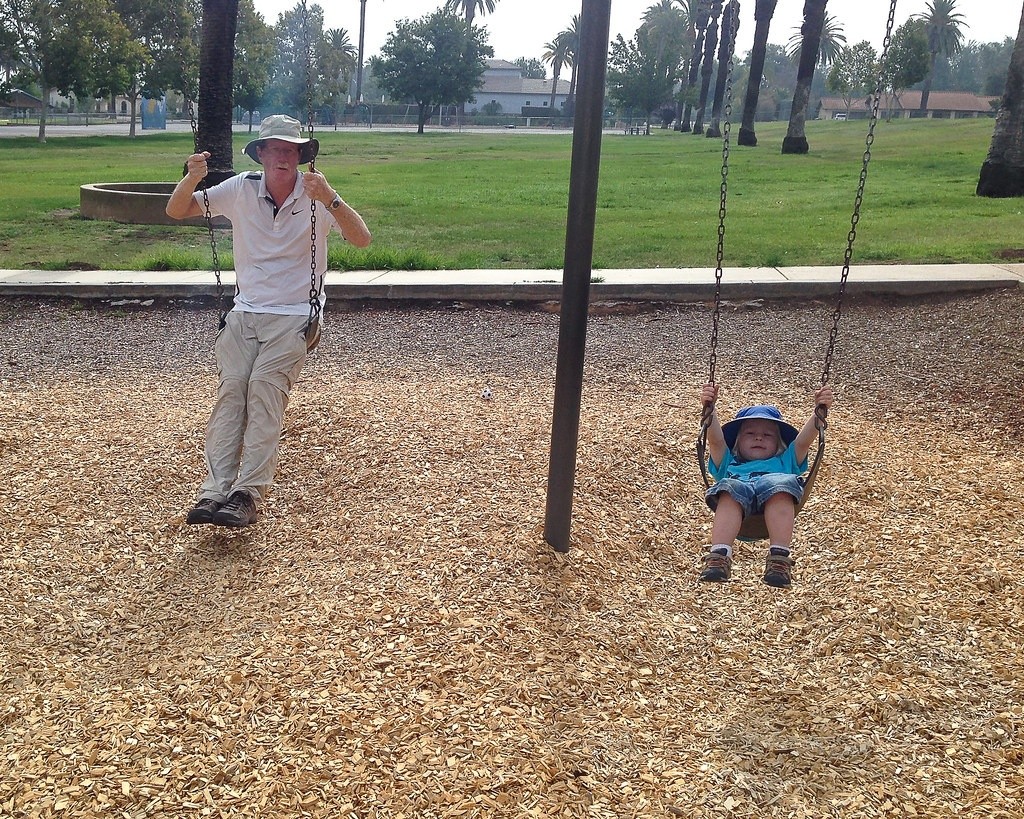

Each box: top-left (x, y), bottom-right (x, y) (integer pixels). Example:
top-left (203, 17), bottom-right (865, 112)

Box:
top-left (166, 0), bottom-right (323, 355)
top-left (694, 0), bottom-right (898, 543)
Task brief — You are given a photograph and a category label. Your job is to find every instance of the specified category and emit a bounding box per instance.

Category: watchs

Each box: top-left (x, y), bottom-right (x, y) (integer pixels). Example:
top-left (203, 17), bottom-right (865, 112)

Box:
top-left (326, 194), bottom-right (341, 212)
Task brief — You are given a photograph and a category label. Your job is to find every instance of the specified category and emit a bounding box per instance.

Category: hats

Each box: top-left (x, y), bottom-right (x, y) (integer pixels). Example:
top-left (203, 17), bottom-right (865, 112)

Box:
top-left (720, 406), bottom-right (799, 452)
top-left (245, 114), bottom-right (320, 165)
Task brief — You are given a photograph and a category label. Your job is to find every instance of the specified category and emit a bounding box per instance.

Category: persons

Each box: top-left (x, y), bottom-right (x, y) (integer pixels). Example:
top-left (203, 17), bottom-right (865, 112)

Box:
top-left (700, 383), bottom-right (834, 588)
top-left (166, 114), bottom-right (371, 528)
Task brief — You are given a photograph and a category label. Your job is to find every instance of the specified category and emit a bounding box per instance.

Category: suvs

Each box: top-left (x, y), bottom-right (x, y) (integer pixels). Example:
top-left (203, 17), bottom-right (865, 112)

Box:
top-left (834, 113), bottom-right (847, 121)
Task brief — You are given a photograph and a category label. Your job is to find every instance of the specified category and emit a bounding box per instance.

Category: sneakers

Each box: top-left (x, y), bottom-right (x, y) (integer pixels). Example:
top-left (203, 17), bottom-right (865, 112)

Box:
top-left (761, 552), bottom-right (796, 588)
top-left (213, 491), bottom-right (258, 528)
top-left (699, 548), bottom-right (732, 581)
top-left (186, 498), bottom-right (224, 525)
